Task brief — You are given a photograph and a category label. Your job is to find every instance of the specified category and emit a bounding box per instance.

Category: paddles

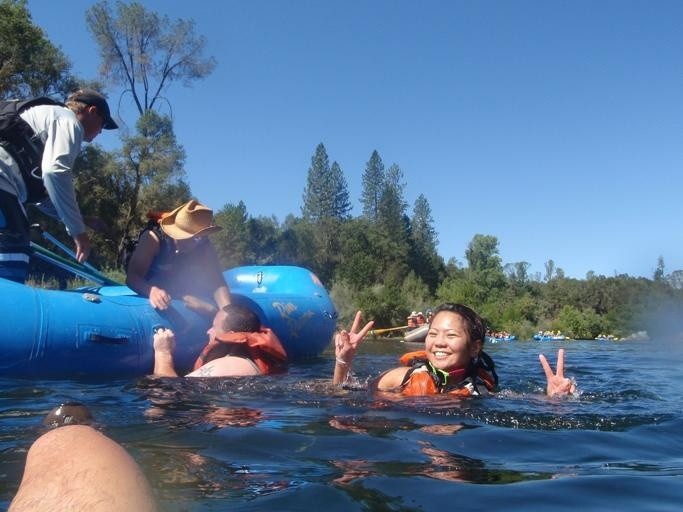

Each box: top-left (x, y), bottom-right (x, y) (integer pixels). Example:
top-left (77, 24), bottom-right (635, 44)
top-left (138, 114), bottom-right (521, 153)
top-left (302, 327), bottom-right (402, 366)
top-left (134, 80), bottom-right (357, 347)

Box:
top-left (365, 326), bottom-right (408, 336)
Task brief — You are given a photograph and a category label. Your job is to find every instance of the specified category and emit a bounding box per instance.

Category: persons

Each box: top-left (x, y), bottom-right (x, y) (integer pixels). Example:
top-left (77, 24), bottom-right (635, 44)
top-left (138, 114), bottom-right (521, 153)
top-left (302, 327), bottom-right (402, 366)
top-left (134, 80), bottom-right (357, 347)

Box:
top-left (147, 392), bottom-right (266, 493)
top-left (145, 291), bottom-right (289, 385)
top-left (0, 76), bottom-right (119, 290)
top-left (323, 299), bottom-right (586, 410)
top-left (325, 409), bottom-right (484, 484)
top-left (5, 423), bottom-right (160, 511)
top-left (122, 195), bottom-right (236, 319)
top-left (392, 307), bottom-right (648, 345)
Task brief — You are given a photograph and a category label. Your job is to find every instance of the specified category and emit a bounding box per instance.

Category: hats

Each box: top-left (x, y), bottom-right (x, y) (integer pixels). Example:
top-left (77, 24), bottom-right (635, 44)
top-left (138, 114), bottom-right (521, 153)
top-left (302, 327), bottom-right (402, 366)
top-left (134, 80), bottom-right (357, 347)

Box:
top-left (160, 199), bottom-right (224, 240)
top-left (63, 89), bottom-right (119, 130)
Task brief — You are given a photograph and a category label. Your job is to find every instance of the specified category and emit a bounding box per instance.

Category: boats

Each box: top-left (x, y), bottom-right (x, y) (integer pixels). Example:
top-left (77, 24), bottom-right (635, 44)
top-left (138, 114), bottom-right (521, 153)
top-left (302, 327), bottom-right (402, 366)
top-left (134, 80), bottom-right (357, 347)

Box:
top-left (0, 266), bottom-right (337, 376)
top-left (533, 334), bottom-right (565, 342)
top-left (403, 324), bottom-right (430, 342)
top-left (595, 336), bottom-right (652, 343)
top-left (484, 335), bottom-right (516, 342)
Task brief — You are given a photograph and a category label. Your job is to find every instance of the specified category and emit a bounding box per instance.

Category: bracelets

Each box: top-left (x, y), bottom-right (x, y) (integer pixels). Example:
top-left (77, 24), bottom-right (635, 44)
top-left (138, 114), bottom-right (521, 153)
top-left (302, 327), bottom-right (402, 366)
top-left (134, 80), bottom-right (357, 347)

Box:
top-left (333, 357), bottom-right (352, 365)
top-left (208, 306), bottom-right (217, 316)
